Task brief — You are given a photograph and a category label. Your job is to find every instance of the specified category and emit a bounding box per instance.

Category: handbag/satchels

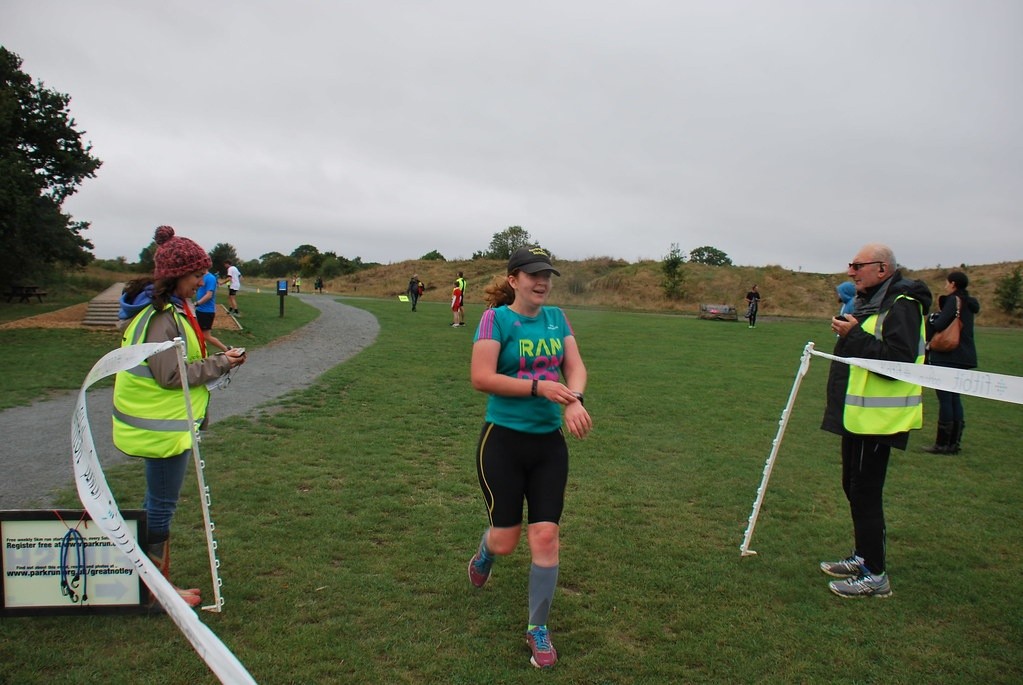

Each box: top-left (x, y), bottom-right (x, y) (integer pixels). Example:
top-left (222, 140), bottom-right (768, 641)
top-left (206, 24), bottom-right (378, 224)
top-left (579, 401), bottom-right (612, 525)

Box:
top-left (928, 295), bottom-right (962, 351)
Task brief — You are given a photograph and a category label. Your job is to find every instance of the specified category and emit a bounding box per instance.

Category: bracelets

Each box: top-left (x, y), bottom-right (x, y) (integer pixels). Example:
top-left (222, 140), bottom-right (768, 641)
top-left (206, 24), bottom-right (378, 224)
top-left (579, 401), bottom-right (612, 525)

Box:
top-left (193, 302), bottom-right (198, 307)
top-left (532, 379), bottom-right (538, 397)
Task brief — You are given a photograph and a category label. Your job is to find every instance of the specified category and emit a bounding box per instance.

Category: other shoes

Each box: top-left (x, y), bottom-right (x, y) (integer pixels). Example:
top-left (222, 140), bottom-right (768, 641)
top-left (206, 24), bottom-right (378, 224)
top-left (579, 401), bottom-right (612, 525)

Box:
top-left (229, 307), bottom-right (240, 317)
top-left (227, 345), bottom-right (233, 349)
top-left (412, 307), bottom-right (416, 312)
top-left (448, 322), bottom-right (466, 328)
top-left (749, 325), bottom-right (756, 328)
top-left (951, 444), bottom-right (958, 454)
top-left (922, 445), bottom-right (952, 454)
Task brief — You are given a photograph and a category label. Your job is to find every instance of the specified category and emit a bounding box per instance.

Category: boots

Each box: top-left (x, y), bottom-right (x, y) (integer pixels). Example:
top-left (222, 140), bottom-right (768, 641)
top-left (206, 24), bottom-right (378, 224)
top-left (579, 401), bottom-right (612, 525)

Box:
top-left (145, 540), bottom-right (202, 608)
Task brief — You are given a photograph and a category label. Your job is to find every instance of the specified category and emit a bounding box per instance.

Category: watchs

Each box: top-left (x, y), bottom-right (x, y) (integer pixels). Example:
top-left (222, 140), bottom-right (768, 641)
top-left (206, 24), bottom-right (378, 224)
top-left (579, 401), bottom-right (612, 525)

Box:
top-left (575, 392), bottom-right (584, 405)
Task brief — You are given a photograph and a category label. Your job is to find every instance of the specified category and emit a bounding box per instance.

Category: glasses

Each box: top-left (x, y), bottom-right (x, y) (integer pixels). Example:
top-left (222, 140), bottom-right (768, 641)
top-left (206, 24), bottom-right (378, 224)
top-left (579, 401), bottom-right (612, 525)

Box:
top-left (849, 261), bottom-right (883, 271)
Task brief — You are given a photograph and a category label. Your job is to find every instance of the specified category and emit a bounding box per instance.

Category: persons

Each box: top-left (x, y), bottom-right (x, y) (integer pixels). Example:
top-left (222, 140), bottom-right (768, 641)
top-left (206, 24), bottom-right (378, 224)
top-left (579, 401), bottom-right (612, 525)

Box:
top-left (451, 281), bottom-right (462, 327)
top-left (820, 244), bottom-right (923, 598)
top-left (111, 225), bottom-right (247, 613)
top-left (209, 259), bottom-right (244, 317)
top-left (449, 271), bottom-right (466, 326)
top-left (192, 269), bottom-right (233, 352)
top-left (407, 274), bottom-right (425, 312)
top-left (836, 281), bottom-right (856, 340)
top-left (471, 248), bottom-right (592, 667)
top-left (924, 270), bottom-right (980, 455)
top-left (745, 285), bottom-right (760, 328)
top-left (292, 274), bottom-right (302, 293)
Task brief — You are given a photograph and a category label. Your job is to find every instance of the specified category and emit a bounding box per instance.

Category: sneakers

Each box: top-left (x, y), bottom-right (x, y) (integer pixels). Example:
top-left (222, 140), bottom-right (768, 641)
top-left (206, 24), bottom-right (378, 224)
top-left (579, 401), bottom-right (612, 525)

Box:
top-left (820, 549), bottom-right (865, 577)
top-left (468, 534), bottom-right (494, 588)
top-left (829, 564), bottom-right (893, 598)
top-left (526, 624), bottom-right (557, 671)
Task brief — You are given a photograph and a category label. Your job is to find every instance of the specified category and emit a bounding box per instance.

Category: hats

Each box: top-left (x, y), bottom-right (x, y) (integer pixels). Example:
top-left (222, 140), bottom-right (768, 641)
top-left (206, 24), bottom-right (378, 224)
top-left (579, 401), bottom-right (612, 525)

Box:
top-left (153, 225), bottom-right (213, 279)
top-left (508, 246), bottom-right (561, 277)
